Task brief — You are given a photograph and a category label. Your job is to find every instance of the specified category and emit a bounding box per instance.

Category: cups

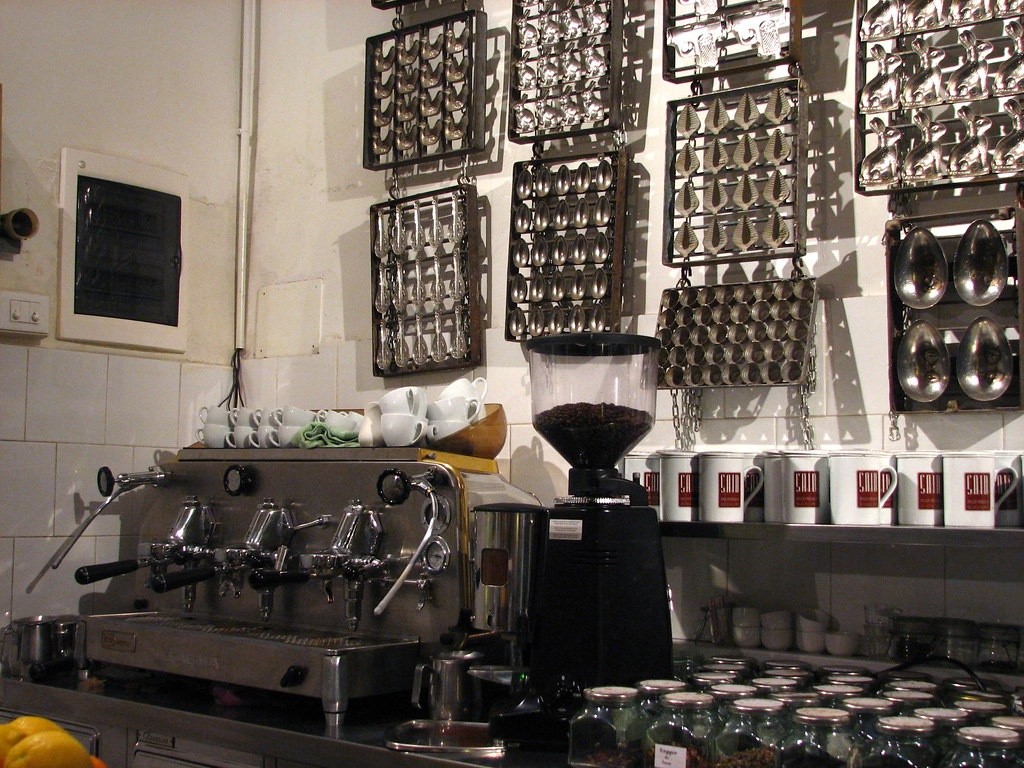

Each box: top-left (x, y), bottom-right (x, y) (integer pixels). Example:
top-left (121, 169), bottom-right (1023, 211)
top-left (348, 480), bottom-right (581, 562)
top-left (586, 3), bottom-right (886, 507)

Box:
top-left (624, 449), bottom-right (1024, 526)
top-left (197, 404), bottom-right (315, 448)
top-left (383, 377), bottom-right (488, 447)
top-left (316, 409), bottom-right (364, 439)
top-left (865, 602), bottom-right (893, 659)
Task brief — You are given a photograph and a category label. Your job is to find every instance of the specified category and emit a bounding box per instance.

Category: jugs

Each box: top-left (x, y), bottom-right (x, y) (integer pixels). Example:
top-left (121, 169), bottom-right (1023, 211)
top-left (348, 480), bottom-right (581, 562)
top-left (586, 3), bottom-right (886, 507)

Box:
top-left (413, 651), bottom-right (487, 725)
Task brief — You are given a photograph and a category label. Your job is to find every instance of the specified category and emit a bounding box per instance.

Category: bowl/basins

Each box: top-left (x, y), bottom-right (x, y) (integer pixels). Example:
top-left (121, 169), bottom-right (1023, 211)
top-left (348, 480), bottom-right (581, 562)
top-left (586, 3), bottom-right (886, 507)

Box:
top-left (825, 630), bottom-right (861, 657)
top-left (431, 403), bottom-right (508, 463)
top-left (734, 610), bottom-right (830, 654)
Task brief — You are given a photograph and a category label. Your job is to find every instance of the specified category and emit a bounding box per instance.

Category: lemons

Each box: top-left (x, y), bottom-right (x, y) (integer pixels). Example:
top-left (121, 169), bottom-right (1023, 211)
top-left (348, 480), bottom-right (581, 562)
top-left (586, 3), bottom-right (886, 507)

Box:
top-left (0, 715), bottom-right (109, 768)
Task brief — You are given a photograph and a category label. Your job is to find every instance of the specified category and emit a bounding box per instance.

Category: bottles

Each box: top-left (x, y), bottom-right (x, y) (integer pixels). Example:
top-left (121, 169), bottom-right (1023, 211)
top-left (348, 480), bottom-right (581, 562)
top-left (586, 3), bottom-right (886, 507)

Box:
top-left (632, 473), bottom-right (640, 484)
top-left (567, 656), bottom-right (1024, 768)
top-left (893, 615), bottom-right (1023, 675)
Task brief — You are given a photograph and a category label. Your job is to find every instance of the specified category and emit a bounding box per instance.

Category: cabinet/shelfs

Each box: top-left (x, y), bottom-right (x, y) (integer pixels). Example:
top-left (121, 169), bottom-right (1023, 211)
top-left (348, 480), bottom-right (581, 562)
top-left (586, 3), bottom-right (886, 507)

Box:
top-left (655, 521), bottom-right (1024, 691)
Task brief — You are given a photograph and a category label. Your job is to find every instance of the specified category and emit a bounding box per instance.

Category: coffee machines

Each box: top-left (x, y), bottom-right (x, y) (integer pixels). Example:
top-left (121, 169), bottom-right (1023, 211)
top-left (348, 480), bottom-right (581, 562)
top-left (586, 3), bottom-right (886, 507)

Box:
top-left (468, 334), bottom-right (678, 742)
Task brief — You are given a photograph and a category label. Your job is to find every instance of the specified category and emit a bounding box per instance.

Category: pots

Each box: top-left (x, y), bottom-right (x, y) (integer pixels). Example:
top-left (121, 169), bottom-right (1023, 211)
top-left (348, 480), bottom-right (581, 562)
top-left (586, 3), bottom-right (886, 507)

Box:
top-left (0, 615), bottom-right (77, 677)
